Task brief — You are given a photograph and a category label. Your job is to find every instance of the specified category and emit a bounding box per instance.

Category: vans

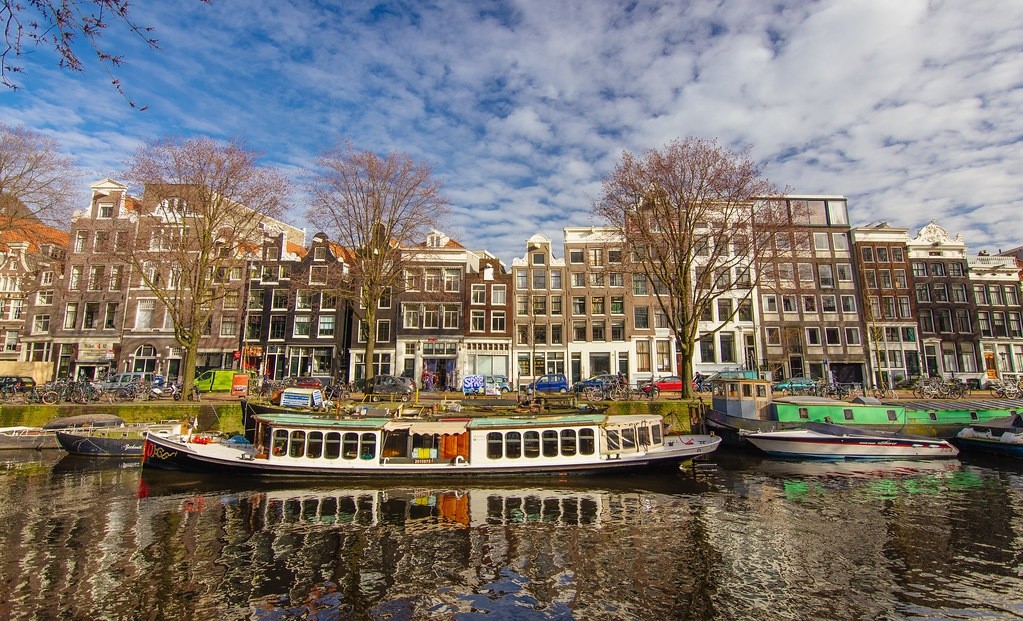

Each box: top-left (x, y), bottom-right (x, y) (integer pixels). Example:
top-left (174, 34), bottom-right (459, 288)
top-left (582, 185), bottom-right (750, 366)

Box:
top-left (704, 370), bottom-right (759, 394)
top-left (99, 372), bottom-right (155, 393)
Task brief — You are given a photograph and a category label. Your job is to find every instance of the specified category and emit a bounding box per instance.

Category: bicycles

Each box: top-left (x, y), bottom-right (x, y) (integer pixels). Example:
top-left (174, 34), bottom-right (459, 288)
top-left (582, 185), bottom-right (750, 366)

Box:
top-left (423, 373), bottom-right (446, 394)
top-left (194, 385), bottom-right (201, 402)
top-left (785, 378), bottom-right (1023, 400)
top-left (692, 382), bottom-right (710, 396)
top-left (326, 381), bottom-right (359, 401)
top-left (1, 379), bottom-right (147, 404)
top-left (585, 379), bottom-right (660, 402)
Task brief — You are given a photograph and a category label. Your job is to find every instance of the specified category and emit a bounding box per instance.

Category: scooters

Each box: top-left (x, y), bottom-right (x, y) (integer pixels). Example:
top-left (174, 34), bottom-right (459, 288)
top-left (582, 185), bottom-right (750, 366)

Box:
top-left (149, 382), bottom-right (180, 401)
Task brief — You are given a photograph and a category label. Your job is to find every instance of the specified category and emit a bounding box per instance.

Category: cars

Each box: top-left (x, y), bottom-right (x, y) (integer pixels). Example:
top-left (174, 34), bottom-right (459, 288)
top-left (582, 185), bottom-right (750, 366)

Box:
top-left (1, 376), bottom-right (37, 393)
top-left (290, 376), bottom-right (324, 393)
top-left (362, 377), bottom-right (416, 393)
top-left (371, 374), bottom-right (413, 402)
top-left (481, 374), bottom-right (511, 394)
top-left (573, 375), bottom-right (628, 393)
top-left (641, 376), bottom-right (698, 394)
top-left (524, 374), bottom-right (569, 394)
top-left (775, 378), bottom-right (815, 392)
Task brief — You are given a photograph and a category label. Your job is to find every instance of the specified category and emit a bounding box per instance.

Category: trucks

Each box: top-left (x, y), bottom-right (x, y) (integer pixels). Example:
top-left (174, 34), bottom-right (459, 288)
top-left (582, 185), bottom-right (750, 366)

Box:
top-left (193, 370), bottom-right (257, 393)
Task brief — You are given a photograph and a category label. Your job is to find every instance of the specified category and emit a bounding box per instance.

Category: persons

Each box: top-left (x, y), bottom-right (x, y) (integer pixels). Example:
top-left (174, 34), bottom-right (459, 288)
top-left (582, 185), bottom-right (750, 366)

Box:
top-left (443, 373), bottom-right (452, 392)
top-left (618, 372), bottom-right (624, 387)
top-left (693, 372), bottom-right (704, 394)
top-left (432, 373), bottom-right (437, 392)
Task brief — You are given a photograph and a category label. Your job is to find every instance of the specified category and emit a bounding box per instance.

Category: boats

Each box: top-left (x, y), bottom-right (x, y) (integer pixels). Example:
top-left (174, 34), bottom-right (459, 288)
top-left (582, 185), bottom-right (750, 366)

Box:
top-left (740, 427), bottom-right (960, 458)
top-left (56, 420), bottom-right (197, 457)
top-left (0, 427), bottom-right (63, 449)
top-left (135, 475), bottom-right (715, 535)
top-left (142, 413), bottom-right (723, 480)
top-left (241, 399), bottom-right (348, 447)
top-left (955, 416), bottom-right (1022, 459)
